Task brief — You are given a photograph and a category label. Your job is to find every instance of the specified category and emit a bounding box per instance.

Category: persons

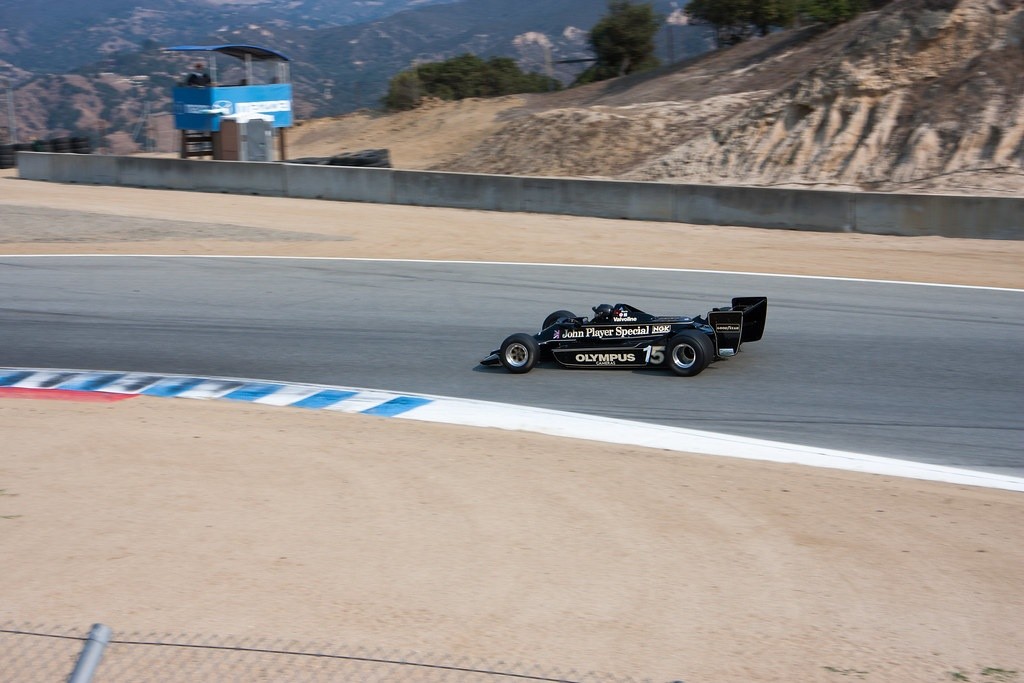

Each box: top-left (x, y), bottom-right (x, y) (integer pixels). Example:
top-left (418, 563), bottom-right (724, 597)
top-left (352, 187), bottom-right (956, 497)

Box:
top-left (187, 64), bottom-right (210, 86)
top-left (583, 304), bottom-right (613, 324)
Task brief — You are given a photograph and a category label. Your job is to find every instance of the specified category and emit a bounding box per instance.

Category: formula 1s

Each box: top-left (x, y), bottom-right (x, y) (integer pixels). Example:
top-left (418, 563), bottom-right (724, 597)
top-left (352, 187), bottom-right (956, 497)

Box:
top-left (480, 293), bottom-right (769, 378)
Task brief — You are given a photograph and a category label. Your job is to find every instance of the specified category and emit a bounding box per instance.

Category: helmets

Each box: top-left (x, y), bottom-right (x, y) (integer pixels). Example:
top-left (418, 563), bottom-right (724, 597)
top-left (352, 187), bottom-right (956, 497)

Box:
top-left (592, 304), bottom-right (613, 320)
